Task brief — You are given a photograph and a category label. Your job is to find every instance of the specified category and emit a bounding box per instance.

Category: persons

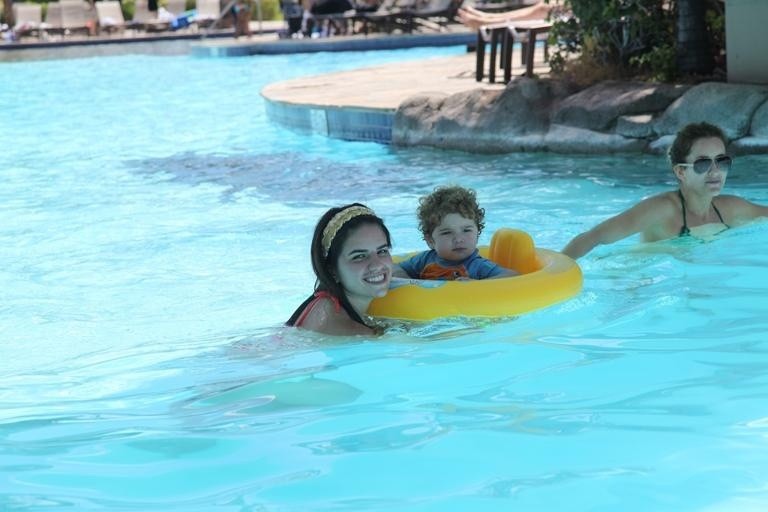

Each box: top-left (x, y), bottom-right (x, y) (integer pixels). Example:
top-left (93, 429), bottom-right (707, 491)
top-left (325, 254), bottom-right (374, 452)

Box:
top-left (388, 182), bottom-right (519, 284)
top-left (226, 0), bottom-right (382, 42)
top-left (456, 1), bottom-right (577, 30)
top-left (560, 120), bottom-right (768, 262)
top-left (283, 202), bottom-right (396, 337)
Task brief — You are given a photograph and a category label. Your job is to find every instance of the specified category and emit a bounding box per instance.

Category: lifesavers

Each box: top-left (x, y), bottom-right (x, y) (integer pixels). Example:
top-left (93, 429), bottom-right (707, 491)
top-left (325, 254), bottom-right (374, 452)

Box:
top-left (367, 228), bottom-right (583, 321)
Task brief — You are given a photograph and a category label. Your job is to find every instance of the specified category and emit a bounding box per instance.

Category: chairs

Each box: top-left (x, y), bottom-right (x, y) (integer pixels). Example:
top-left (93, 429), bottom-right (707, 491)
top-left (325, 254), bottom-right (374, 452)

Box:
top-left (311, 0), bottom-right (584, 84)
top-left (0, 1), bottom-right (224, 41)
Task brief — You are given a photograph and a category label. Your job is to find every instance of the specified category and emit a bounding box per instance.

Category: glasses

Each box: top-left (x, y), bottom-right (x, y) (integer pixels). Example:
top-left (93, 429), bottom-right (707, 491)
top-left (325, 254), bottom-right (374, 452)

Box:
top-left (673, 156), bottom-right (733, 175)
